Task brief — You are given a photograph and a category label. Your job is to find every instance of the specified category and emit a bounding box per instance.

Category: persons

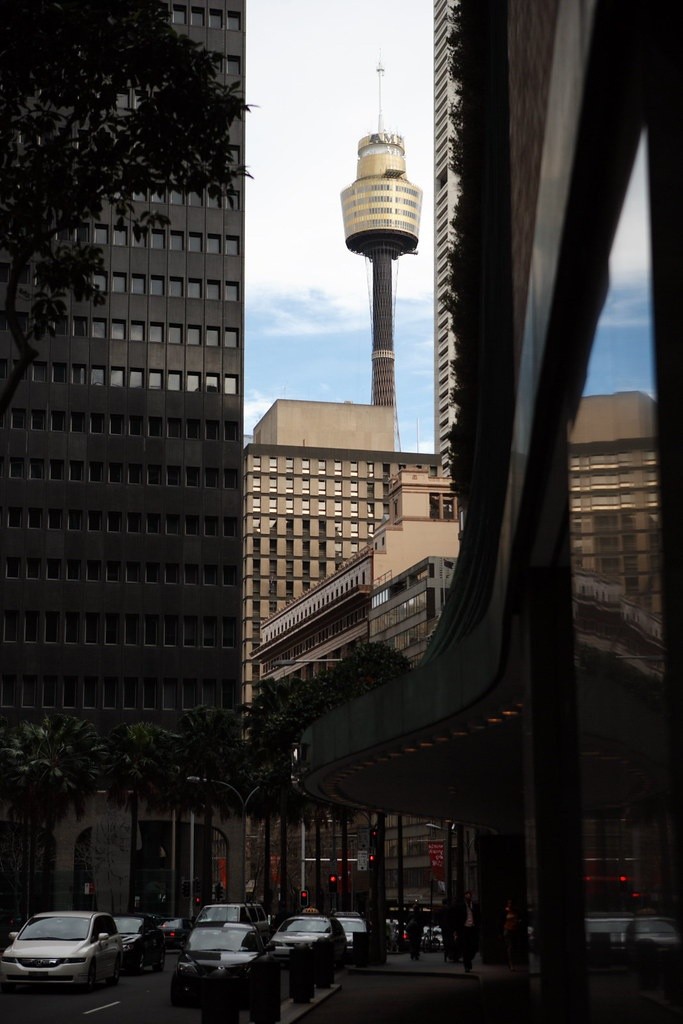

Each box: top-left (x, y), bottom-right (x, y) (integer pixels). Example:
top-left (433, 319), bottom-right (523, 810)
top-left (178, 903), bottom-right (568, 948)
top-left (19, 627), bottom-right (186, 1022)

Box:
top-left (388, 894), bottom-right (525, 973)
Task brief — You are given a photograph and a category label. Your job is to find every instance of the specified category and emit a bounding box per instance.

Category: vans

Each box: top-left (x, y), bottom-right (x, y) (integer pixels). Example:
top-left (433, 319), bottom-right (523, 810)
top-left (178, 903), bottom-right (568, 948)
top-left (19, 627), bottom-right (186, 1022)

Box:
top-left (194, 903), bottom-right (269, 946)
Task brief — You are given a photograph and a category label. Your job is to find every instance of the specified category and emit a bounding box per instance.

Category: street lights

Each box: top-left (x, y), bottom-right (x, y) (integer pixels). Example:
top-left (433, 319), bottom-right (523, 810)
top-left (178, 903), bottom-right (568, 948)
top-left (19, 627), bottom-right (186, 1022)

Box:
top-left (427, 824), bottom-right (474, 892)
top-left (186, 777), bottom-right (261, 903)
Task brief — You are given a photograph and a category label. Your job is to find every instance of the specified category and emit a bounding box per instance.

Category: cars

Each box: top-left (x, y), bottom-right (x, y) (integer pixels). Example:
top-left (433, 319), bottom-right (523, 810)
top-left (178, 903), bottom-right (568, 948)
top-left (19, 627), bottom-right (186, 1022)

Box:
top-left (266, 907), bottom-right (348, 970)
top-left (113, 917), bottom-right (166, 970)
top-left (171, 921), bottom-right (276, 1006)
top-left (333, 911), bottom-right (367, 950)
top-left (583, 916), bottom-right (683, 956)
top-left (157, 918), bottom-right (193, 948)
top-left (0, 910), bottom-right (123, 991)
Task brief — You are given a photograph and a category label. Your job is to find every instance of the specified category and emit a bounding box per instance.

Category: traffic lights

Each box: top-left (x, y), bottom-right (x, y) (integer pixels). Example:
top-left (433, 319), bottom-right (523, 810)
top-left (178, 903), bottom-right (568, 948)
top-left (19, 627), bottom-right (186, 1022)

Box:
top-left (368, 829), bottom-right (376, 870)
top-left (300, 891), bottom-right (309, 906)
top-left (194, 896), bottom-right (201, 907)
top-left (328, 873), bottom-right (337, 894)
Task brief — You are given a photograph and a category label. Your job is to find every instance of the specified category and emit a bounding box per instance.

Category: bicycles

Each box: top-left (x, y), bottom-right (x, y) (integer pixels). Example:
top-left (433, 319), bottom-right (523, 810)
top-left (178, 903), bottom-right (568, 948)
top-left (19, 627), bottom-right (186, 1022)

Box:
top-left (423, 931), bottom-right (440, 953)
